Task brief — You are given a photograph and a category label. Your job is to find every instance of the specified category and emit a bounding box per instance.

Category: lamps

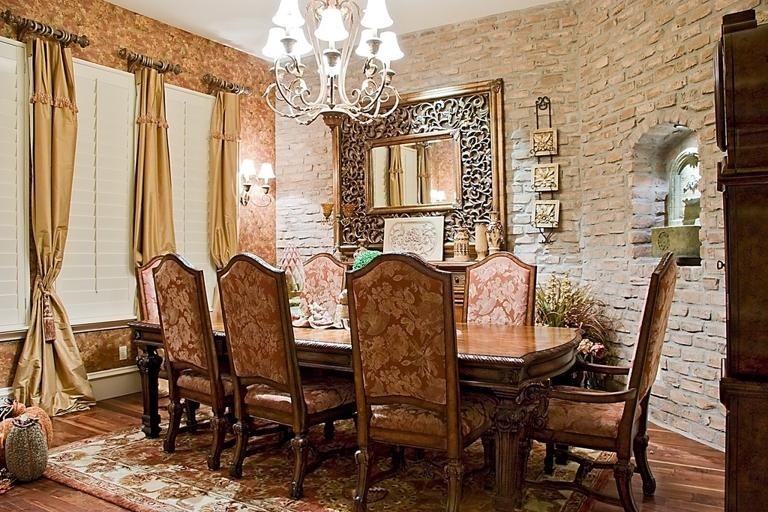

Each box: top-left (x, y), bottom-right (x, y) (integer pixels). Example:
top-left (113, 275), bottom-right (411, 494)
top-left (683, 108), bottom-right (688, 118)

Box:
top-left (238, 159), bottom-right (276, 207)
top-left (320, 203), bottom-right (355, 226)
top-left (261, 0), bottom-right (404, 133)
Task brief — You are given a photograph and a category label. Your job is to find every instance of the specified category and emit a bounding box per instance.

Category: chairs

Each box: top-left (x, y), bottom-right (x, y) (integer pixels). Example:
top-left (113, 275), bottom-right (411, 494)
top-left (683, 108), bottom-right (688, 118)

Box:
top-left (302, 252), bottom-right (347, 321)
top-left (216, 252), bottom-right (356, 500)
top-left (461, 252), bottom-right (537, 326)
top-left (152, 253), bottom-right (235, 467)
top-left (345, 253), bottom-right (498, 512)
top-left (527, 253), bottom-right (677, 510)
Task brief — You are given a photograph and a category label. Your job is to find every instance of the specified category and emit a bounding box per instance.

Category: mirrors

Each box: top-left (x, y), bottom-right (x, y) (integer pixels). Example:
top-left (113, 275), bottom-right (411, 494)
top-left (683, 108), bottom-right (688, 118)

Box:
top-left (364, 128), bottom-right (463, 215)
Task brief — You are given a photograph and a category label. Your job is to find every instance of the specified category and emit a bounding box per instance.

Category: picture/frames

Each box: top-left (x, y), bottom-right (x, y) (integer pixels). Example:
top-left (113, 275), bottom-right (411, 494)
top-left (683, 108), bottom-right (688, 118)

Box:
top-left (331, 78), bottom-right (507, 255)
top-left (531, 200), bottom-right (560, 228)
top-left (529, 128), bottom-right (558, 156)
top-left (383, 216), bottom-right (445, 262)
top-left (530, 163), bottom-right (559, 191)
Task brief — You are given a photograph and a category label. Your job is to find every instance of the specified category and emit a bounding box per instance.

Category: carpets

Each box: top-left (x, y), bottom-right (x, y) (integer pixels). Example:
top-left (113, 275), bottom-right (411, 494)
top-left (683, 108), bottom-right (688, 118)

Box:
top-left (43, 408), bottom-right (619, 512)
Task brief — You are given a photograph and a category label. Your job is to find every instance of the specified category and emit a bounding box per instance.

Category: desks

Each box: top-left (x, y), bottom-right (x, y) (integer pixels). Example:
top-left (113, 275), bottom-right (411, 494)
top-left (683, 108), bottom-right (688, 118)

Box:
top-left (128, 307), bottom-right (586, 512)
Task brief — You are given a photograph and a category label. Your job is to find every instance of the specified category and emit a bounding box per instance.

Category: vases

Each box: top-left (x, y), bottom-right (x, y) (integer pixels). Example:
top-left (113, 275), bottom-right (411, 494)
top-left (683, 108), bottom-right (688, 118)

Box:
top-left (454, 227), bottom-right (469, 261)
top-left (486, 210), bottom-right (503, 255)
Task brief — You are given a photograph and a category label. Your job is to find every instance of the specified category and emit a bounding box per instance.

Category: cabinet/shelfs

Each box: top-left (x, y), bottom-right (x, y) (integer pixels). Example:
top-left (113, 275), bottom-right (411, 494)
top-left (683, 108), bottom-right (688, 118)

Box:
top-left (715, 9), bottom-right (768, 512)
